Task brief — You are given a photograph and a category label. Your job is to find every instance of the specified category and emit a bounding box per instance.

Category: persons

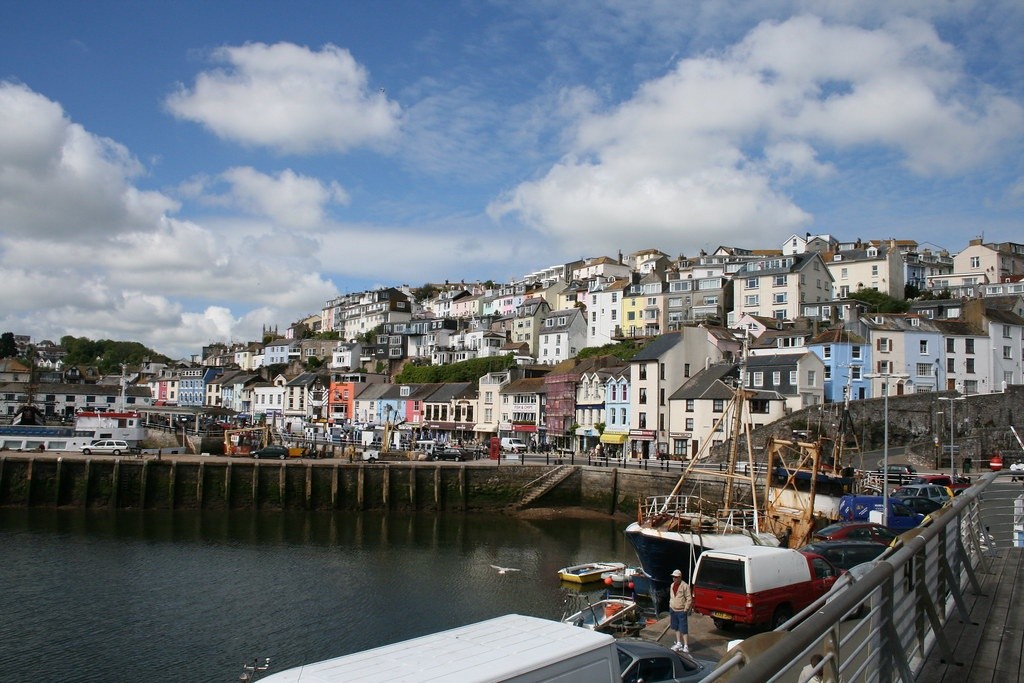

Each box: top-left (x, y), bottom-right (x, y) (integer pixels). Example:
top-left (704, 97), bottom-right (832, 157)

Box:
top-left (455, 435), bottom-right (626, 461)
top-left (797, 654), bottom-right (824, 683)
top-left (348, 444), bottom-right (355, 464)
top-left (839, 564), bottom-right (848, 575)
top-left (668, 569), bottom-right (692, 653)
top-left (1010, 459), bottom-right (1024, 483)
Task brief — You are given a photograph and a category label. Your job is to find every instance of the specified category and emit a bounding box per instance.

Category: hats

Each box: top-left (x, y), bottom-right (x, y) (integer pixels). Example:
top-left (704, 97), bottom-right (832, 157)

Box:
top-left (670, 570), bottom-right (682, 576)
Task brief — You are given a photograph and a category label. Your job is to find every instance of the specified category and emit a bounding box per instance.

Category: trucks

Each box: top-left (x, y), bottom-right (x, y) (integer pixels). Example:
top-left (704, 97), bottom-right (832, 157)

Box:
top-left (250, 613), bottom-right (622, 682)
top-left (500, 438), bottom-right (526, 453)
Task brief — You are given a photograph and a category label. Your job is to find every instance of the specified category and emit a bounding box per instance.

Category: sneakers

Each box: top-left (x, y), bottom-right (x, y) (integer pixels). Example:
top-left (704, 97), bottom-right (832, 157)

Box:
top-left (682, 647), bottom-right (689, 653)
top-left (671, 644), bottom-right (683, 651)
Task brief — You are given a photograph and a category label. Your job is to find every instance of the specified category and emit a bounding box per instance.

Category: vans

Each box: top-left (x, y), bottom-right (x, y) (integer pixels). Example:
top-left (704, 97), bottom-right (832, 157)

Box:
top-left (838, 493), bottom-right (933, 534)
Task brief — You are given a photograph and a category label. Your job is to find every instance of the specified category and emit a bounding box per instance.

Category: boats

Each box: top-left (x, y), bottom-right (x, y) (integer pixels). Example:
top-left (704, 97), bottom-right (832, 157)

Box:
top-left (601, 566), bottom-right (669, 599)
top-left (0, 360), bottom-right (187, 454)
top-left (557, 562), bottom-right (627, 584)
top-left (561, 594), bottom-right (637, 636)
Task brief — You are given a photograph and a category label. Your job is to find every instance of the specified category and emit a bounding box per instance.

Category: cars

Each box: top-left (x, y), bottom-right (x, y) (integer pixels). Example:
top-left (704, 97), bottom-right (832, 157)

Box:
top-left (79, 440), bottom-right (130, 456)
top-left (811, 521), bottom-right (900, 545)
top-left (249, 448), bottom-right (474, 462)
top-left (797, 541), bottom-right (887, 571)
top-left (891, 496), bottom-right (942, 515)
top-left (615, 637), bottom-right (718, 683)
top-left (892, 485), bottom-right (950, 504)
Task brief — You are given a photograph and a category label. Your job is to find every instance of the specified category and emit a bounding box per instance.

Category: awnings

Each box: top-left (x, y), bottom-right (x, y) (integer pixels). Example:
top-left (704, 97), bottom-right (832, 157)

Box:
top-left (599, 433), bottom-right (625, 444)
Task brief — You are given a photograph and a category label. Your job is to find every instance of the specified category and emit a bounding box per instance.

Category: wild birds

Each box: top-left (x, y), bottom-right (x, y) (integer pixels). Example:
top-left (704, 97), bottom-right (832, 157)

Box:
top-left (489, 564), bottom-right (522, 575)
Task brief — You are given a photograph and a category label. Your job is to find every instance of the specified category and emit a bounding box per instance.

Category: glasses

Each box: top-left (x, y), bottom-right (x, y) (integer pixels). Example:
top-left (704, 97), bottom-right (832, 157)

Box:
top-left (671, 576), bottom-right (678, 578)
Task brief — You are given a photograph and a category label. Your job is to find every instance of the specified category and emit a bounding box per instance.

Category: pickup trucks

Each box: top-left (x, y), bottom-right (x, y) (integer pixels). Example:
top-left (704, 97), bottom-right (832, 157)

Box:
top-left (909, 475), bottom-right (971, 497)
top-left (691, 546), bottom-right (864, 636)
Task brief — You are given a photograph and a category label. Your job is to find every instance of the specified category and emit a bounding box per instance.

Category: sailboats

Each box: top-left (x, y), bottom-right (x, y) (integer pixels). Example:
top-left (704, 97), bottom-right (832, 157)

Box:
top-left (624, 322), bottom-right (884, 586)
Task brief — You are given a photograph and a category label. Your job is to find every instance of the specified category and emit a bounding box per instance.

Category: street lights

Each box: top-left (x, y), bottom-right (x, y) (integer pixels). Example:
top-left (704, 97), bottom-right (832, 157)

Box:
top-left (863, 373), bottom-right (911, 527)
top-left (938, 397), bottom-right (966, 484)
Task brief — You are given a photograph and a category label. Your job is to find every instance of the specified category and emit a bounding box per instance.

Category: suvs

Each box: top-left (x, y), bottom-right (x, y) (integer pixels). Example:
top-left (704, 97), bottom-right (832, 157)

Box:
top-left (866, 464), bottom-right (917, 485)
